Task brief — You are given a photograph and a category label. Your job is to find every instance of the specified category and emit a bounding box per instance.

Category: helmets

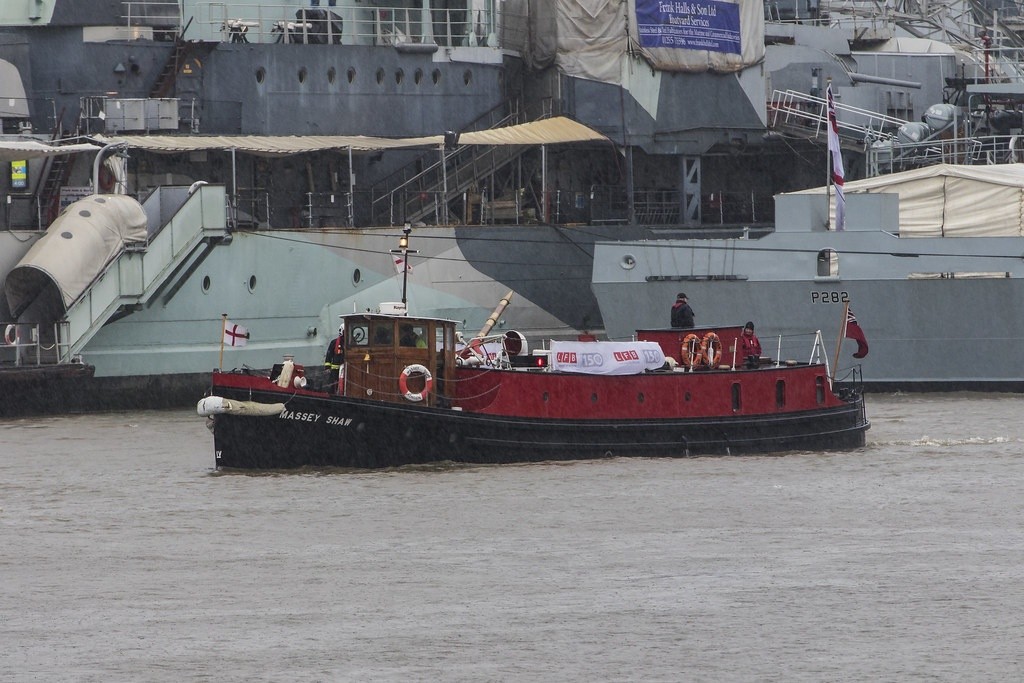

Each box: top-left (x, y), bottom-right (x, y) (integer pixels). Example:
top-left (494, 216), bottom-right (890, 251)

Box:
top-left (339, 322), bottom-right (346, 336)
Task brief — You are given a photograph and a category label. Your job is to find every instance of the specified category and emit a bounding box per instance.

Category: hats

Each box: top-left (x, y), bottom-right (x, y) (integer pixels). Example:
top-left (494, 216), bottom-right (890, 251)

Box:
top-left (677, 293), bottom-right (689, 300)
top-left (746, 322), bottom-right (754, 330)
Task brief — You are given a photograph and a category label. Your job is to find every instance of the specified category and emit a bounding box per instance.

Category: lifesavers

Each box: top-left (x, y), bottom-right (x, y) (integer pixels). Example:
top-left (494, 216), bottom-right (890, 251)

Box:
top-left (338, 363), bottom-right (345, 397)
top-left (398, 362), bottom-right (434, 403)
top-left (680, 332), bottom-right (724, 371)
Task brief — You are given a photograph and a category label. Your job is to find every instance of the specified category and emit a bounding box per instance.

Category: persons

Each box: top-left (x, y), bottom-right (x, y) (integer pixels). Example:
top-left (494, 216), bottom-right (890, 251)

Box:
top-left (738, 321), bottom-right (763, 371)
top-left (670, 293), bottom-right (695, 328)
top-left (324, 323), bottom-right (345, 394)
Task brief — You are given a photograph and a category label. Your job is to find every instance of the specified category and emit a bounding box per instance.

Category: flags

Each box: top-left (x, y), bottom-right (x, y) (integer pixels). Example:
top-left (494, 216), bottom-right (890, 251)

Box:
top-left (224, 321), bottom-right (248, 347)
top-left (827, 86), bottom-right (846, 232)
top-left (392, 255), bottom-right (413, 274)
top-left (843, 307), bottom-right (868, 359)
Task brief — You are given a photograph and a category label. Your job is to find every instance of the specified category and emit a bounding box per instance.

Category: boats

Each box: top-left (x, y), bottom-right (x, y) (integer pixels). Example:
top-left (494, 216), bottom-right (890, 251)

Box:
top-left (198, 222), bottom-right (871, 468)
top-left (1, 0), bottom-right (1024, 412)
